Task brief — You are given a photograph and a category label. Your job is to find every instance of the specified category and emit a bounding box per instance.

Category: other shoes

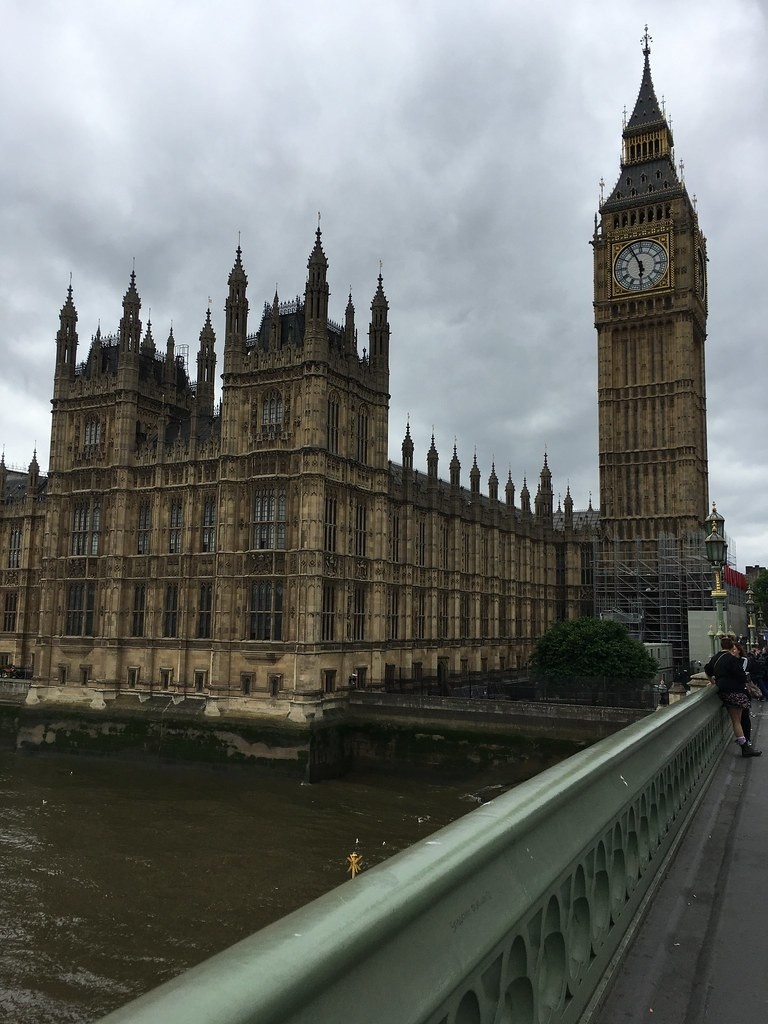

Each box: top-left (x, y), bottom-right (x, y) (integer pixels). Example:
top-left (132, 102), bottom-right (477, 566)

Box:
top-left (761, 698), bottom-right (767, 702)
top-left (740, 747), bottom-right (762, 758)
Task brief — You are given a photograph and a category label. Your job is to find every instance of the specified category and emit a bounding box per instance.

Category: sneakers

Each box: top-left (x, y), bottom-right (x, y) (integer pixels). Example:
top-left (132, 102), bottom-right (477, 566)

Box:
top-left (735, 736), bottom-right (750, 745)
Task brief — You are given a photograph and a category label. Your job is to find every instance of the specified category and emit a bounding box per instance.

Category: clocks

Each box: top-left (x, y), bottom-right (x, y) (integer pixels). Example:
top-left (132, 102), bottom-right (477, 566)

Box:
top-left (612, 238), bottom-right (670, 292)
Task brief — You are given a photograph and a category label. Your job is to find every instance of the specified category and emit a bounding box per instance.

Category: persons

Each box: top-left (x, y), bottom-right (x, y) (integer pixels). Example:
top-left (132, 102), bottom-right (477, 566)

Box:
top-left (746, 645), bottom-right (768, 695)
top-left (704, 638), bottom-right (762, 757)
top-left (732, 644), bottom-right (752, 745)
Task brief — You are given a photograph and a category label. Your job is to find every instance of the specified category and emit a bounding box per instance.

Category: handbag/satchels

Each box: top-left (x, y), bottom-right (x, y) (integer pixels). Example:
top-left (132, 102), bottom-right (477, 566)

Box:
top-left (746, 681), bottom-right (763, 699)
